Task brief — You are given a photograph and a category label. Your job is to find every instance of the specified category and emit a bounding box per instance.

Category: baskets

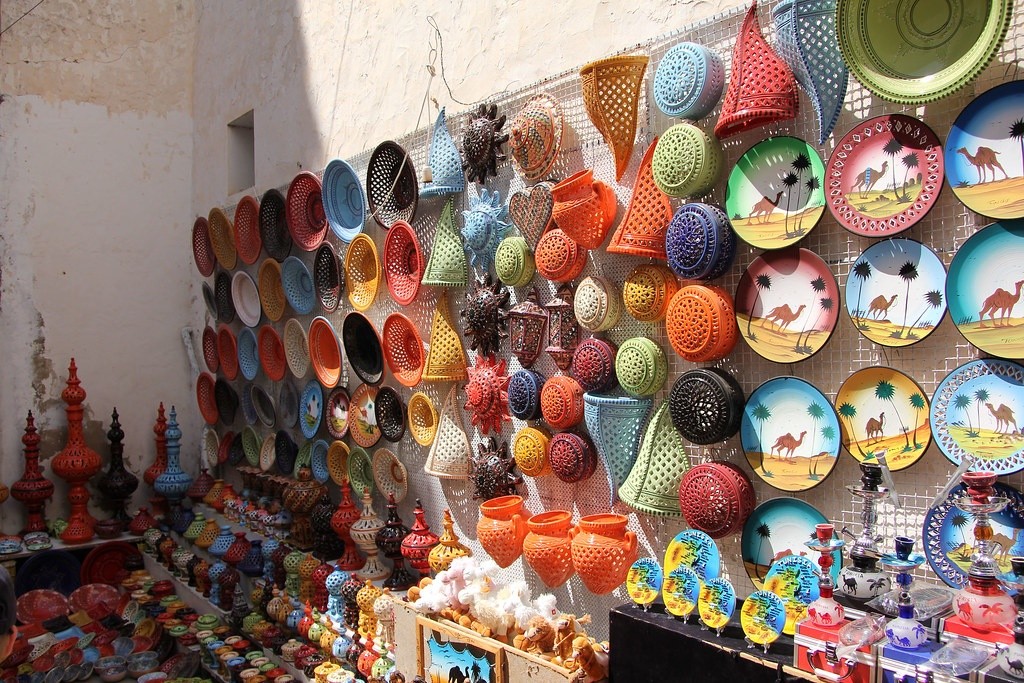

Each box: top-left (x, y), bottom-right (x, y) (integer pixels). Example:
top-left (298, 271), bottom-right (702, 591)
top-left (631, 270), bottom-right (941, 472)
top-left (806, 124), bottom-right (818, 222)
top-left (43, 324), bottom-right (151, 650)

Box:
top-left (194, 141), bottom-right (439, 506)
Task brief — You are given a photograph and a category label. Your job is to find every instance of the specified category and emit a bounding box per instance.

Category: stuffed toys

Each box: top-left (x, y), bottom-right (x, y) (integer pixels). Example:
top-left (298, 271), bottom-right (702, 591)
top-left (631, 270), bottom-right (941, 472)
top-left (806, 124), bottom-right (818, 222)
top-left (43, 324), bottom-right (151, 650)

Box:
top-left (408, 557), bottom-right (609, 683)
